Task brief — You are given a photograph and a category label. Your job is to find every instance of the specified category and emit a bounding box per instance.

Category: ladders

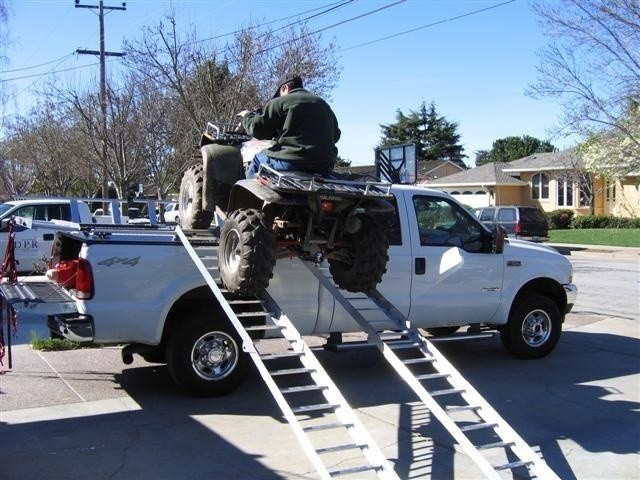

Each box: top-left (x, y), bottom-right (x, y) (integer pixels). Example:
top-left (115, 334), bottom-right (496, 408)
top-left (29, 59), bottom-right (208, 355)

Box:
top-left (287, 244), bottom-right (561, 480)
top-left (13, 195), bottom-right (180, 230)
top-left (176, 224), bottom-right (402, 480)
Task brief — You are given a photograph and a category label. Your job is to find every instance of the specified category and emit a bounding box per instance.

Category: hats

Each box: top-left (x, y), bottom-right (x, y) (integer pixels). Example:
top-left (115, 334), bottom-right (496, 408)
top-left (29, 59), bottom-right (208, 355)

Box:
top-left (272, 72), bottom-right (302, 98)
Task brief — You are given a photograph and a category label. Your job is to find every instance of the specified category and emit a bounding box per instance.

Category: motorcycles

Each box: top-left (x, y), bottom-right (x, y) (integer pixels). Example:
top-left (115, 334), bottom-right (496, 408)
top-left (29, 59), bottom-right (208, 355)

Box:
top-left (165, 104), bottom-right (404, 306)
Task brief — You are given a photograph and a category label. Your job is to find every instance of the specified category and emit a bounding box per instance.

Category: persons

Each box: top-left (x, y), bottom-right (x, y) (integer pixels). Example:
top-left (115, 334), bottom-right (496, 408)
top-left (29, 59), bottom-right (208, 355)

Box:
top-left (237, 71), bottom-right (341, 223)
